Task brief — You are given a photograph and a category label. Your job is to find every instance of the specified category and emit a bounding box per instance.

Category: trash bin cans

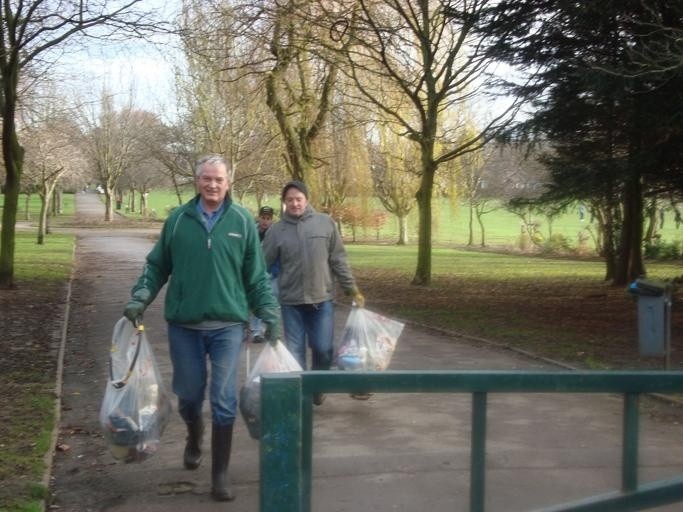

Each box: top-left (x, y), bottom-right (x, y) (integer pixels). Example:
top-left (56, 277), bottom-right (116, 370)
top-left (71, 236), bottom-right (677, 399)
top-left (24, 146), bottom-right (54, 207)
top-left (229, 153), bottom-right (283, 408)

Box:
top-left (627, 277), bottom-right (673, 371)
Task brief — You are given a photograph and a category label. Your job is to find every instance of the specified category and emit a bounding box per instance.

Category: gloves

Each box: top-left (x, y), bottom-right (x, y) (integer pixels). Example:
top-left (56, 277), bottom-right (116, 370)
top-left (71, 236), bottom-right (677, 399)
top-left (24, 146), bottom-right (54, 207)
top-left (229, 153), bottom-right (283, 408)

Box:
top-left (351, 292), bottom-right (366, 308)
top-left (122, 302), bottom-right (145, 323)
top-left (263, 327), bottom-right (284, 348)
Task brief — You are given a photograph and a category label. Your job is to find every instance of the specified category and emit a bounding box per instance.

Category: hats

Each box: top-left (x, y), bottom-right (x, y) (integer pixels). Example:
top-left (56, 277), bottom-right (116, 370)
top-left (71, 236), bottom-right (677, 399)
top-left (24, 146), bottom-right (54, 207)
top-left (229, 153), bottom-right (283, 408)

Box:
top-left (281, 180), bottom-right (309, 204)
top-left (259, 205), bottom-right (274, 219)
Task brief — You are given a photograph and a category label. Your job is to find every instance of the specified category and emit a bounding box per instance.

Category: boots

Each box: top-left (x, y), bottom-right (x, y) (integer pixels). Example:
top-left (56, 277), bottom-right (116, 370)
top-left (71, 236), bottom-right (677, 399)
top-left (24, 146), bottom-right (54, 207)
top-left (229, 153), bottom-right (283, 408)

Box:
top-left (178, 403), bottom-right (208, 471)
top-left (211, 419), bottom-right (234, 502)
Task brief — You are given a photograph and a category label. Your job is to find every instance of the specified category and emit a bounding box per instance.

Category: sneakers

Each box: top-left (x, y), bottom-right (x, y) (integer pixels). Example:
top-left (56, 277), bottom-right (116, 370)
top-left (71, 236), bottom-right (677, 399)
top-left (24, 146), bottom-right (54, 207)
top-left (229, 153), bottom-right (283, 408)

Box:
top-left (313, 392), bottom-right (327, 407)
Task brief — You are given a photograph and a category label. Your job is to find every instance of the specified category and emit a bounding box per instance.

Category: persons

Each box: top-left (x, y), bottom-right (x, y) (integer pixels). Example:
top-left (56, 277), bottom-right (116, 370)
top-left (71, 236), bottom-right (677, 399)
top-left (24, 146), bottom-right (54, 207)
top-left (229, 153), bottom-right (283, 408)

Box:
top-left (249, 205), bottom-right (280, 344)
top-left (258, 182), bottom-right (366, 407)
top-left (123, 154), bottom-right (282, 503)
